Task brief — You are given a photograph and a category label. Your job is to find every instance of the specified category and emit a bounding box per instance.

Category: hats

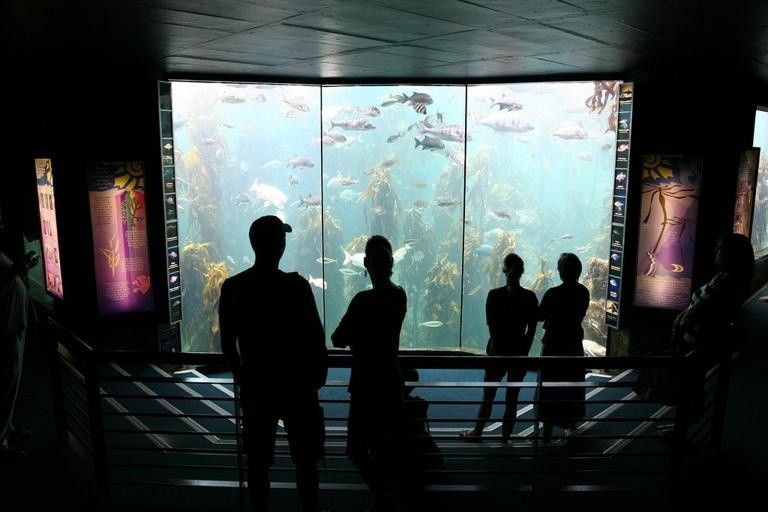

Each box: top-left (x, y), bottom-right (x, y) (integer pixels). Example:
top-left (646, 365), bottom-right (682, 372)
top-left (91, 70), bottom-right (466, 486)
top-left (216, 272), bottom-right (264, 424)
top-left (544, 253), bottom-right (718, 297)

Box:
top-left (246, 214), bottom-right (293, 235)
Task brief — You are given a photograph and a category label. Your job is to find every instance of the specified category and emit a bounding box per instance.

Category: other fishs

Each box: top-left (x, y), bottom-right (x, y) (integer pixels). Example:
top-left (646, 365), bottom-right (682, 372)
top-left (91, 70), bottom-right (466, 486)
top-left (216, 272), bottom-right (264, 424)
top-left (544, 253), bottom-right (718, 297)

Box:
top-left (169, 81), bottom-right (615, 328)
top-left (608, 79), bottom-right (632, 298)
top-left (160, 134), bottom-right (182, 312)
top-left (582, 339), bottom-right (606, 356)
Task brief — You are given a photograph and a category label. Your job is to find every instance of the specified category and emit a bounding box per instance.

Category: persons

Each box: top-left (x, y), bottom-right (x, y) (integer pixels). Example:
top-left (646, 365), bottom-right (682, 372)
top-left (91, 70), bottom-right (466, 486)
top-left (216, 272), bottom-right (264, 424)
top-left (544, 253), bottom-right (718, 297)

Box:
top-left (524, 252), bottom-right (590, 445)
top-left (0, 213), bottom-right (40, 451)
top-left (402, 369), bottom-right (450, 485)
top-left (671, 233), bottom-right (755, 352)
top-left (458, 253), bottom-right (539, 444)
top-left (218, 217), bottom-right (329, 512)
top-left (332, 236), bottom-right (407, 511)
